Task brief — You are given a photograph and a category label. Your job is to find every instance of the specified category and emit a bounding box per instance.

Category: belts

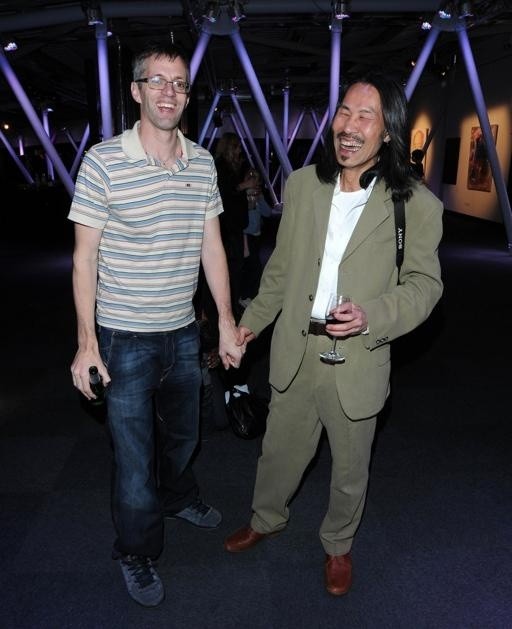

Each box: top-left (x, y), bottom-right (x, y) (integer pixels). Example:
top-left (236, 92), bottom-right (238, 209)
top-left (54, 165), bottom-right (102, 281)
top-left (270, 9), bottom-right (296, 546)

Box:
top-left (307, 321), bottom-right (333, 336)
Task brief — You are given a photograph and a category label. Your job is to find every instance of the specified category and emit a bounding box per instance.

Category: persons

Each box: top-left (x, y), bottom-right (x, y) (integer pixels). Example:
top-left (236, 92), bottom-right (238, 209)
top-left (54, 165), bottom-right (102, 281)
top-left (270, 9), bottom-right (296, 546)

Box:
top-left (213, 132), bottom-right (252, 307)
top-left (412, 149), bottom-right (424, 172)
top-left (67, 41), bottom-right (247, 608)
top-left (232, 71), bottom-right (444, 594)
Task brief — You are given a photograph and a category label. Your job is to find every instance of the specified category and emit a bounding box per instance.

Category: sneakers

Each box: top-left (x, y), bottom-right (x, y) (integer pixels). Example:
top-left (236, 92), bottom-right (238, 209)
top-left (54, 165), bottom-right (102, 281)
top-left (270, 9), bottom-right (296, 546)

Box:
top-left (166, 497), bottom-right (223, 530)
top-left (120, 554), bottom-right (165, 606)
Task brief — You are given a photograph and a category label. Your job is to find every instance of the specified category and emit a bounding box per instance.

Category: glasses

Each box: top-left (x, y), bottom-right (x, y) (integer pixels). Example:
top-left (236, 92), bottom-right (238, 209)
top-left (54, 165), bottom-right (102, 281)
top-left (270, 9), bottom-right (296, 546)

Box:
top-left (134, 76), bottom-right (191, 93)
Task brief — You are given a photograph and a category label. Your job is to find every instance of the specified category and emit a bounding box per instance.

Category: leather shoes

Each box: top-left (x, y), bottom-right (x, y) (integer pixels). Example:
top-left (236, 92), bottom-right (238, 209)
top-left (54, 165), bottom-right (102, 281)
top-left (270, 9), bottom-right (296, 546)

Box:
top-left (224, 524), bottom-right (278, 551)
top-left (325, 552), bottom-right (352, 595)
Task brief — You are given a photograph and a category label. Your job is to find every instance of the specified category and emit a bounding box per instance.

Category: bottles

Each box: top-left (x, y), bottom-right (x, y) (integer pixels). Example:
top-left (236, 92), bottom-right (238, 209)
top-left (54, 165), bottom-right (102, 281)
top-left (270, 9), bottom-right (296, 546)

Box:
top-left (86, 364), bottom-right (109, 410)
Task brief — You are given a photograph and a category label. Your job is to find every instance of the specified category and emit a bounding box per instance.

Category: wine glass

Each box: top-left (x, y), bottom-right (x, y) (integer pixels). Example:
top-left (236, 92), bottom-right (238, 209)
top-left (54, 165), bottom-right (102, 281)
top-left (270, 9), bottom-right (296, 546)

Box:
top-left (315, 292), bottom-right (357, 367)
